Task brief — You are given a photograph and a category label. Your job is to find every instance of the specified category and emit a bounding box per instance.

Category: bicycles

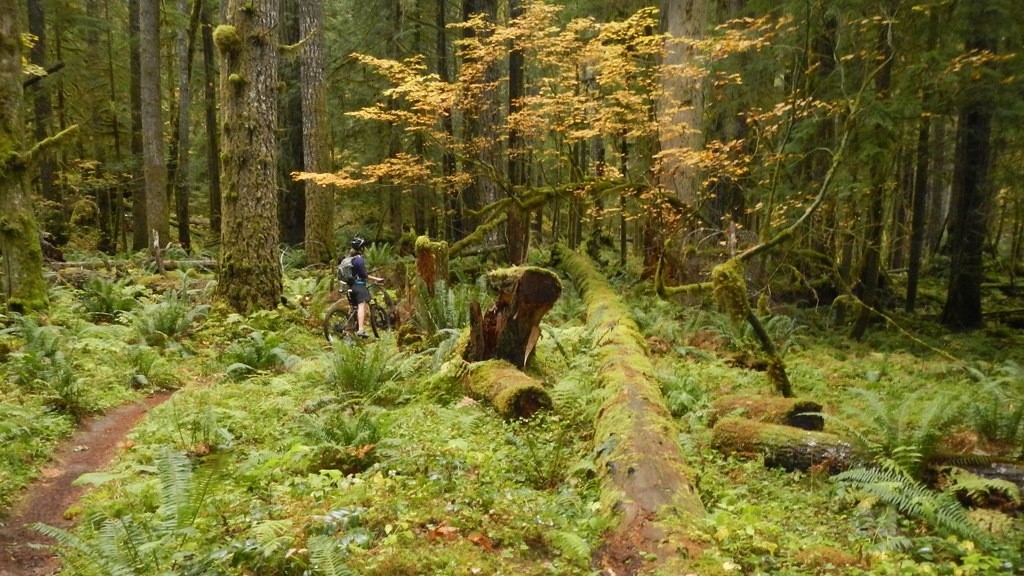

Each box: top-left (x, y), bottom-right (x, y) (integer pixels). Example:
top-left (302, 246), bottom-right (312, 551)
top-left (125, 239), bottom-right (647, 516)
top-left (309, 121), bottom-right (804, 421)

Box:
top-left (324, 279), bottom-right (389, 346)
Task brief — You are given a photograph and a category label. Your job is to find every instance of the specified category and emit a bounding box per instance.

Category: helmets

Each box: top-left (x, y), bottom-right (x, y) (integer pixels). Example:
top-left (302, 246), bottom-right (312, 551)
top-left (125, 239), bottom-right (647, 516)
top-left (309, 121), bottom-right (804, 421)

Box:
top-left (351, 238), bottom-right (365, 251)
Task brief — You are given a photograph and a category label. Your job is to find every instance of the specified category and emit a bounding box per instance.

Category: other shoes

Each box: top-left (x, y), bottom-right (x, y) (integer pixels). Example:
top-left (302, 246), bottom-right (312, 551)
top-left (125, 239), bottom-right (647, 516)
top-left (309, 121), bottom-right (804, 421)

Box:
top-left (357, 330), bottom-right (370, 338)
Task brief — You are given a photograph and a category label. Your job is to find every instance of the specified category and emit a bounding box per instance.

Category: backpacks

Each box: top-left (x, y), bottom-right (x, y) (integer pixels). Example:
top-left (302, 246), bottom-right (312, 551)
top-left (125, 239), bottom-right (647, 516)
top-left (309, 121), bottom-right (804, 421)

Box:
top-left (337, 255), bottom-right (362, 282)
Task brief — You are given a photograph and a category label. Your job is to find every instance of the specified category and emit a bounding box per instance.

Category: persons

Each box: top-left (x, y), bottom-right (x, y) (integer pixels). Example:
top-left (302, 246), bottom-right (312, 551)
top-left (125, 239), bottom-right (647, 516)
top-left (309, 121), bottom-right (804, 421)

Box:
top-left (336, 237), bottom-right (384, 338)
